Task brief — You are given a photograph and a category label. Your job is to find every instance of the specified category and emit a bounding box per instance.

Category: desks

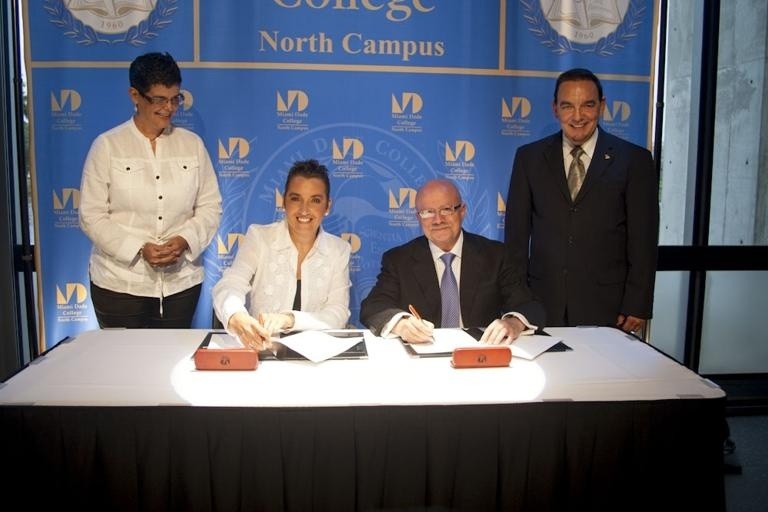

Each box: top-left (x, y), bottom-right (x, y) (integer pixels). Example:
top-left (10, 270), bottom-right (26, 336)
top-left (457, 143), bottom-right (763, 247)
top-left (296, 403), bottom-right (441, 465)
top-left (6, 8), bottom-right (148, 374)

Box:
top-left (0, 328), bottom-right (727, 510)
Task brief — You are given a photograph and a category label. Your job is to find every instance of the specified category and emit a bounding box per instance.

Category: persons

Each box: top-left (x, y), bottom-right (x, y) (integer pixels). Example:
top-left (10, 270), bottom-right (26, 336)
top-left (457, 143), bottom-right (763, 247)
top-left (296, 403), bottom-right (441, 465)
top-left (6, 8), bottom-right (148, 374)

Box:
top-left (360, 178), bottom-right (547, 345)
top-left (212, 160), bottom-right (352, 351)
top-left (504, 69), bottom-right (660, 333)
top-left (77, 52), bottom-right (223, 328)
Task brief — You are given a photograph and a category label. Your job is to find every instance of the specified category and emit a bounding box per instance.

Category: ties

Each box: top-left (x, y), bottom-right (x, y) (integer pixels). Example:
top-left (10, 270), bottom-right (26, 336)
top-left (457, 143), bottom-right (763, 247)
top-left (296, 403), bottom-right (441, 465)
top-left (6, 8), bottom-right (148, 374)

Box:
top-left (438, 252), bottom-right (460, 328)
top-left (567, 145), bottom-right (585, 203)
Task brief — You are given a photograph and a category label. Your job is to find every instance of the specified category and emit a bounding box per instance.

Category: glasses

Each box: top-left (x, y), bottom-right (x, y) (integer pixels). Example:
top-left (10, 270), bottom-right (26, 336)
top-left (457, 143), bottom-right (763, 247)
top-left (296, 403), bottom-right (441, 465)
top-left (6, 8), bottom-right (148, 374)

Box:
top-left (418, 204), bottom-right (461, 220)
top-left (139, 91), bottom-right (185, 105)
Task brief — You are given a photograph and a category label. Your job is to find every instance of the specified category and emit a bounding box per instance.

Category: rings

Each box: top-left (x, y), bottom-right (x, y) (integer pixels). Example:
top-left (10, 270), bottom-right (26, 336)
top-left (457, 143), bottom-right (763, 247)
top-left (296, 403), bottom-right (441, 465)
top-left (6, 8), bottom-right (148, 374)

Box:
top-left (153, 264), bottom-right (157, 269)
top-left (248, 343), bottom-right (255, 348)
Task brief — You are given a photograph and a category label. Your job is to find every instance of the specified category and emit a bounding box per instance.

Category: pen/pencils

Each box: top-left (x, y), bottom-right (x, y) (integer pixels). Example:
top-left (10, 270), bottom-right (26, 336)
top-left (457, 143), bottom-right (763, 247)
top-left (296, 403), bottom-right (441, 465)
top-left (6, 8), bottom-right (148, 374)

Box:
top-left (259, 314), bottom-right (266, 350)
top-left (409, 304), bottom-right (435, 341)
